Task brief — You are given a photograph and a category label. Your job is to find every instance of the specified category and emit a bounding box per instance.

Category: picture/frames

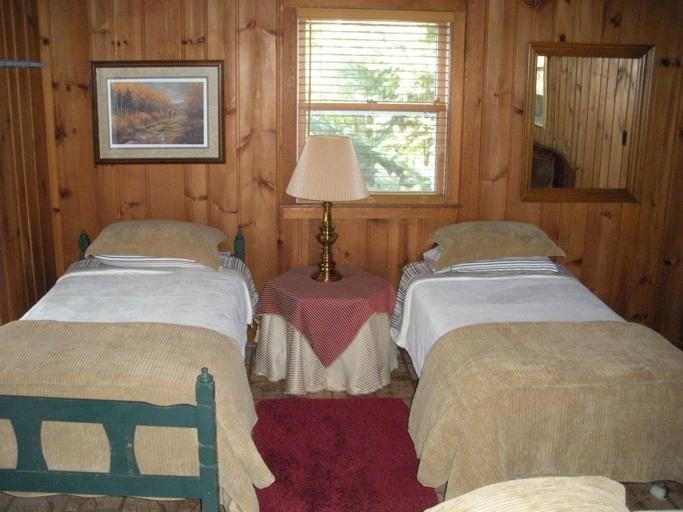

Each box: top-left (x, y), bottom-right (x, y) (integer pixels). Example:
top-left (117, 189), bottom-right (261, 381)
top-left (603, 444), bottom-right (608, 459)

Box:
top-left (91, 60), bottom-right (227, 162)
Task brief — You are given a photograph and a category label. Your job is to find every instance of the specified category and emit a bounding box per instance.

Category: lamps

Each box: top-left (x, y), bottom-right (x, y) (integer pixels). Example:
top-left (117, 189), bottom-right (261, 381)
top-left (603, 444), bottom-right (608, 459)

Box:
top-left (285, 135), bottom-right (369, 283)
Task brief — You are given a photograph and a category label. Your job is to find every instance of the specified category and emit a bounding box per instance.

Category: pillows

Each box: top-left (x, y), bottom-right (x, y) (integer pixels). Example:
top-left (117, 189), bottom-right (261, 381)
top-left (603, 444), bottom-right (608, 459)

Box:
top-left (424, 220), bottom-right (566, 274)
top-left (84, 219), bottom-right (228, 273)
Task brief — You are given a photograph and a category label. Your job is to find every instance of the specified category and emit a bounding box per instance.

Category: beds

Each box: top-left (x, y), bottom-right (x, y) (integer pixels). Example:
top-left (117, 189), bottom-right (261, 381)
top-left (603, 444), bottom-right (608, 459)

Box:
top-left (0, 228), bottom-right (257, 512)
top-left (404, 262), bottom-right (683, 500)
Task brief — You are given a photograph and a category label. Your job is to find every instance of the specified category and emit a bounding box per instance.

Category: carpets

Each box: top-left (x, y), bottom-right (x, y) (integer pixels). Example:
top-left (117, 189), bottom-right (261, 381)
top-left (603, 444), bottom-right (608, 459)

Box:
top-left (249, 397), bottom-right (439, 512)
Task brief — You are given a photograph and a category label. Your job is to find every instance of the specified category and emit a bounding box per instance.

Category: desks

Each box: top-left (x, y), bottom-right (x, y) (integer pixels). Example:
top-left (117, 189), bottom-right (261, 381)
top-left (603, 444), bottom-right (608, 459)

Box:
top-left (266, 267), bottom-right (390, 391)
